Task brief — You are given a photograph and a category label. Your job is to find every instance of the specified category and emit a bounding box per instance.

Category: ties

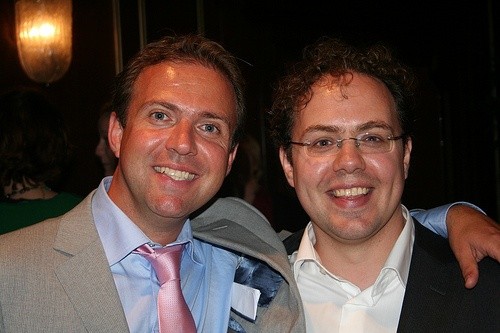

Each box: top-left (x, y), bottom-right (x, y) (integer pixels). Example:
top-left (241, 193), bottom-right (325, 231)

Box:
top-left (131, 242), bottom-right (199, 332)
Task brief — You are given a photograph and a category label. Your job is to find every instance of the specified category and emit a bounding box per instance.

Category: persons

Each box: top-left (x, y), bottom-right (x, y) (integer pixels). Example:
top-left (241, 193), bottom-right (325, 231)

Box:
top-left (276, 39), bottom-right (499, 332)
top-left (93, 100), bottom-right (125, 176)
top-left (2, 150), bottom-right (64, 200)
top-left (1, 33), bottom-right (499, 333)
top-left (1, 92), bottom-right (82, 237)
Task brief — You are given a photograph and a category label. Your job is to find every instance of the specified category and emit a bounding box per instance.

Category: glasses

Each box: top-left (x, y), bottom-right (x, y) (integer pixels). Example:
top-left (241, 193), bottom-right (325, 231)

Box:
top-left (288, 132), bottom-right (407, 159)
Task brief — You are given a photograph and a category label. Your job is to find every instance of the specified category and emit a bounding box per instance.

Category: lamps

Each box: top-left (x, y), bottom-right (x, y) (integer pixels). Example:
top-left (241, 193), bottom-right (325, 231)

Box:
top-left (14, 0), bottom-right (73, 83)
